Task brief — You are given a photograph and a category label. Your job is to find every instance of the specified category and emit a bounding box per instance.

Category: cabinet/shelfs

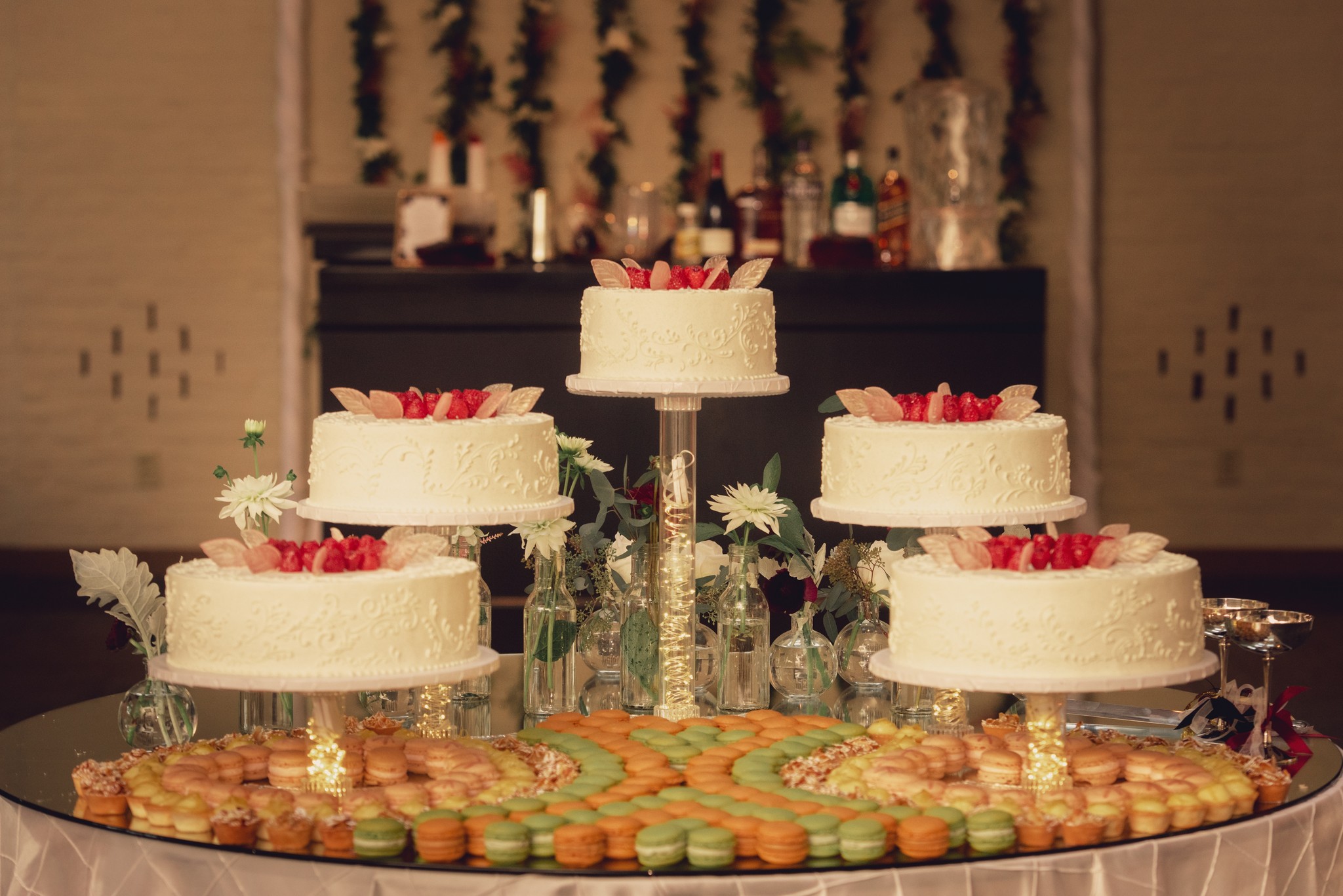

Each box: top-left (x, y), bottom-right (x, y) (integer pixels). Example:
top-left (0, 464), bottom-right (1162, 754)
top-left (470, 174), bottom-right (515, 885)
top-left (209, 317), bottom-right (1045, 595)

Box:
top-left (316, 260), bottom-right (1047, 653)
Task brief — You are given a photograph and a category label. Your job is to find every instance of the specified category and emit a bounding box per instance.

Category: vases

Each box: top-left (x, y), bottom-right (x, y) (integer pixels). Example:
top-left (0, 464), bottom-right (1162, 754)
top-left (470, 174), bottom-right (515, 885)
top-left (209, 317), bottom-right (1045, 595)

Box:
top-left (716, 544), bottom-right (771, 711)
top-left (890, 684), bottom-right (935, 715)
top-left (620, 542), bottom-right (663, 714)
top-left (238, 690), bottom-right (293, 732)
top-left (695, 619), bottom-right (721, 695)
top-left (769, 610), bottom-right (839, 704)
top-left (358, 689), bottom-right (418, 729)
top-left (448, 541), bottom-right (492, 702)
top-left (577, 589), bottom-right (627, 683)
top-left (833, 601), bottom-right (890, 688)
top-left (117, 657), bottom-right (198, 753)
top-left (444, 675), bottom-right (891, 738)
top-left (522, 545), bottom-right (576, 715)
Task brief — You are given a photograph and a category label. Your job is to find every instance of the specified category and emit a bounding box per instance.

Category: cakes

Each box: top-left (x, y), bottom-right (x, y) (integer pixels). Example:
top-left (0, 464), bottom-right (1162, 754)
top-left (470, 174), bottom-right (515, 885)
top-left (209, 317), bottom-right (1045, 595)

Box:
top-left (164, 526), bottom-right (484, 675)
top-left (817, 383), bottom-right (1070, 513)
top-left (578, 254), bottom-right (777, 381)
top-left (305, 382), bottom-right (559, 513)
top-left (888, 522), bottom-right (1205, 676)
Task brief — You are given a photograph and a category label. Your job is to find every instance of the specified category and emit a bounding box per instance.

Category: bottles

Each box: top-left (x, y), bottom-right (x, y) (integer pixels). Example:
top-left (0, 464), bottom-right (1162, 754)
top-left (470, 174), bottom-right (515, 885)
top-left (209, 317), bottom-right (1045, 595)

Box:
top-left (428, 121), bottom-right (912, 268)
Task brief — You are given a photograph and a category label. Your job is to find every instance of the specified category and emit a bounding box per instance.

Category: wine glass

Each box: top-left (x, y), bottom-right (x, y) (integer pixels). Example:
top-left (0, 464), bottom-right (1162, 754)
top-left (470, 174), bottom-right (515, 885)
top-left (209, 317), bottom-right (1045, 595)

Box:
top-left (1224, 609), bottom-right (1314, 764)
top-left (1201, 597), bottom-right (1270, 730)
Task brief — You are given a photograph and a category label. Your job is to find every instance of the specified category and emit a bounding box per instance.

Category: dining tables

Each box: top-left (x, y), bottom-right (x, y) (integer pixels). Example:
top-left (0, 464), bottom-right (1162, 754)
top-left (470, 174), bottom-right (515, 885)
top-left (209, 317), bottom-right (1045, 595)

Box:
top-left (3, 695), bottom-right (1343, 896)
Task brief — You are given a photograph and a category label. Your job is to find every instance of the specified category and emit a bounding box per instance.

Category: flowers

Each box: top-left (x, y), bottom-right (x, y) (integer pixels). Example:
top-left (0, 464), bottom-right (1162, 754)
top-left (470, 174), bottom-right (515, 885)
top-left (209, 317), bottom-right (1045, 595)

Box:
top-left (213, 418), bottom-right (295, 720)
top-left (69, 546), bottom-right (195, 744)
top-left (448, 394), bottom-right (923, 697)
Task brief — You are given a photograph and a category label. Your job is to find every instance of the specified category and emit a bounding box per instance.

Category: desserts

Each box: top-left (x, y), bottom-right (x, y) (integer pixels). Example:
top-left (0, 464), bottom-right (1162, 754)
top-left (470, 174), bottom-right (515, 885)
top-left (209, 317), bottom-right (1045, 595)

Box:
top-left (70, 709), bottom-right (1292, 865)
